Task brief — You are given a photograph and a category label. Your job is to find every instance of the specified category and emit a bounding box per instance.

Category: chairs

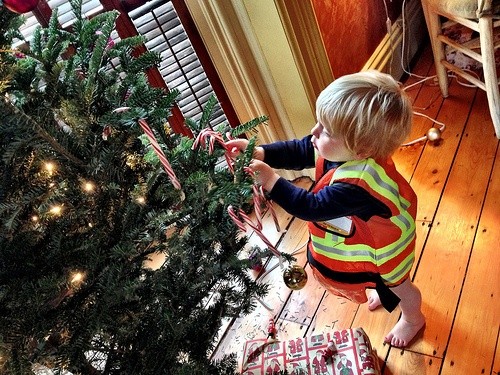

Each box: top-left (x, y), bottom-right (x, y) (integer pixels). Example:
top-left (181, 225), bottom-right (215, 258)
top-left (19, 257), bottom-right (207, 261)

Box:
top-left (421, 0), bottom-right (500, 140)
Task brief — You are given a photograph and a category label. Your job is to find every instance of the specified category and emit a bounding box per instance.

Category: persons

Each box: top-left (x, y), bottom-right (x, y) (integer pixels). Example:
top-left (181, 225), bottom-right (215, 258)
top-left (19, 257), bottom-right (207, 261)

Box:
top-left (226, 71), bottom-right (425, 346)
